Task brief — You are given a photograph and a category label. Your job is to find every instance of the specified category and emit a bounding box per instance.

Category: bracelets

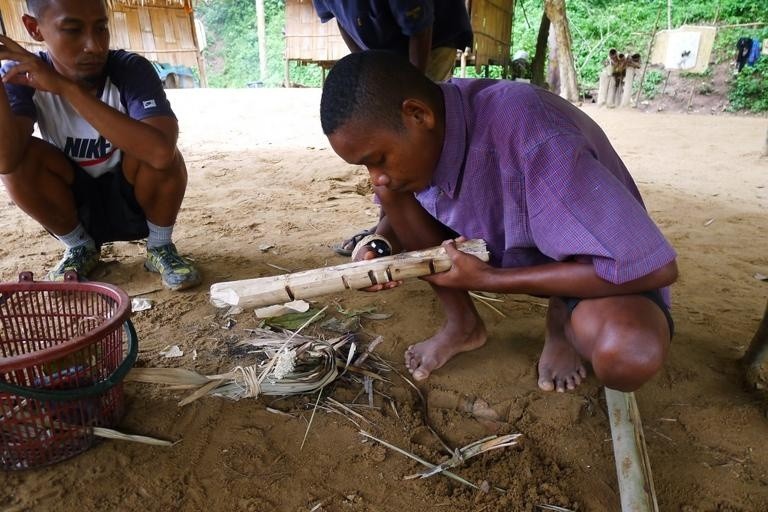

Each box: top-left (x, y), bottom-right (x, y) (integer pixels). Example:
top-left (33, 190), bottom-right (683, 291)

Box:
top-left (370, 240), bottom-right (390, 256)
top-left (351, 234), bottom-right (392, 262)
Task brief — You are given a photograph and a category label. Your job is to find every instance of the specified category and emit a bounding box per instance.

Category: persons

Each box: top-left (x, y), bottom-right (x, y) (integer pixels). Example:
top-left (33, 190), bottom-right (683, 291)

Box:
top-left (0, 0), bottom-right (204, 291)
top-left (512, 50), bottom-right (530, 74)
top-left (317, 50), bottom-right (680, 395)
top-left (700, 301), bottom-right (768, 378)
top-left (311, 1), bottom-right (477, 262)
top-left (603, 49), bottom-right (643, 87)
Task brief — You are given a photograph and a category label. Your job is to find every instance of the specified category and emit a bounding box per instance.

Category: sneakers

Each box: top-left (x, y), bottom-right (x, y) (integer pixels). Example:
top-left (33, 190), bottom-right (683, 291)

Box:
top-left (45, 243), bottom-right (101, 297)
top-left (142, 243), bottom-right (202, 289)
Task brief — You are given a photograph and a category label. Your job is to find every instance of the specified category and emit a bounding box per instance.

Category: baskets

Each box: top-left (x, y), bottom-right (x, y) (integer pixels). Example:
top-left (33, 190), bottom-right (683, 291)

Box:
top-left (0, 268), bottom-right (138, 475)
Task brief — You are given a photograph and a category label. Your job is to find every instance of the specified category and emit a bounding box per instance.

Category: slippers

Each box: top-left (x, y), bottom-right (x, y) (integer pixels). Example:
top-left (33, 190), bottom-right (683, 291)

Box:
top-left (336, 230), bottom-right (372, 257)
